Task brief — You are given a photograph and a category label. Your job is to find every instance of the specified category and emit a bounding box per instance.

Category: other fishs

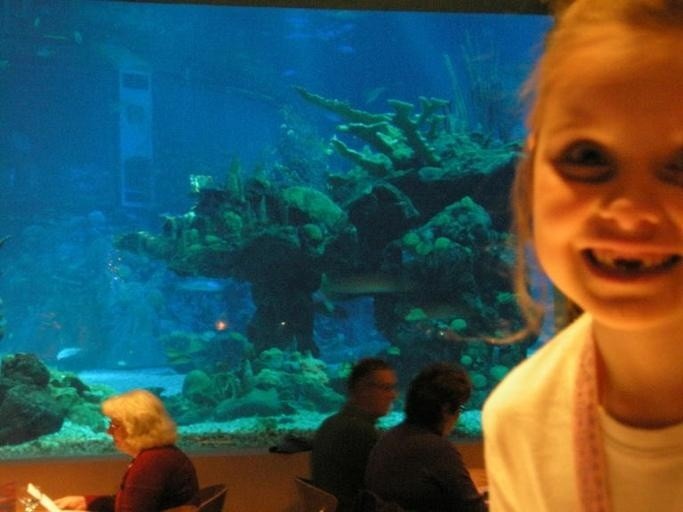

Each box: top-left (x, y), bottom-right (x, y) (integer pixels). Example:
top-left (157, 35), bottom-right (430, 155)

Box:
top-left (175, 278), bottom-right (225, 295)
top-left (55, 347), bottom-right (83, 361)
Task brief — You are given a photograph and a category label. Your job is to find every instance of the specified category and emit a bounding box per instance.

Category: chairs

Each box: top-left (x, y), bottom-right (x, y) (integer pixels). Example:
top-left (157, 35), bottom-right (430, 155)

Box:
top-left (171, 478), bottom-right (340, 512)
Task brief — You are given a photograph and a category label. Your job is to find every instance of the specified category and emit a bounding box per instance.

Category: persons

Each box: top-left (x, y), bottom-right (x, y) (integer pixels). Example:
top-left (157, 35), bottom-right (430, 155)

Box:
top-left (359, 359), bottom-right (488, 512)
top-left (47, 390), bottom-right (200, 511)
top-left (313, 356), bottom-right (398, 510)
top-left (470, 1), bottom-right (683, 510)
